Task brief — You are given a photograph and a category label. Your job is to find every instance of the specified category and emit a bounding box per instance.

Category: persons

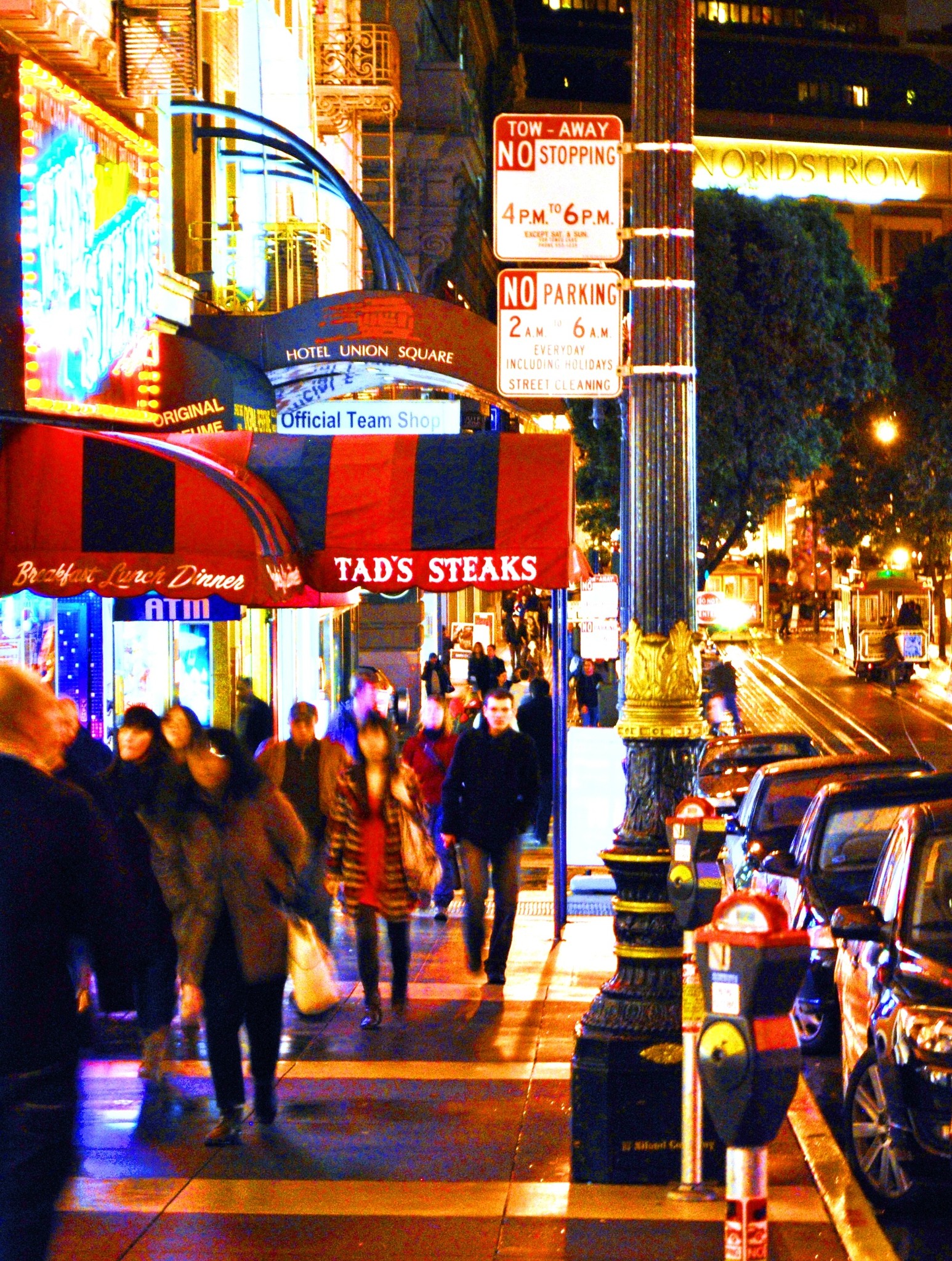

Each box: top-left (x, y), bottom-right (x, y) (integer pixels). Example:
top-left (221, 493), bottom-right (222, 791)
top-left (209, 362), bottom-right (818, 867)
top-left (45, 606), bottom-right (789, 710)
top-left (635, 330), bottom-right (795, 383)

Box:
top-left (253, 702), bottom-right (352, 952)
top-left (93, 706), bottom-right (183, 1081)
top-left (54, 585), bottom-right (559, 824)
top-left (0, 660), bottom-right (139, 1261)
top-left (575, 659), bottom-right (601, 727)
top-left (326, 719), bottom-right (427, 1028)
top-left (401, 695), bottom-right (461, 923)
top-left (778, 592), bottom-right (791, 637)
top-left (899, 601), bottom-right (923, 627)
top-left (151, 729), bottom-right (309, 1145)
top-left (881, 622), bottom-right (901, 696)
top-left (442, 689), bottom-right (538, 985)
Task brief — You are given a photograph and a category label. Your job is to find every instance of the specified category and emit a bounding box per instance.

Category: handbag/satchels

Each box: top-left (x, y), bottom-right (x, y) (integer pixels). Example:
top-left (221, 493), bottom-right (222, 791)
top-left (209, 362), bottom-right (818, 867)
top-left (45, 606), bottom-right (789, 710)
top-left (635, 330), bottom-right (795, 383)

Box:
top-left (397, 808), bottom-right (443, 895)
top-left (284, 912), bottom-right (340, 1015)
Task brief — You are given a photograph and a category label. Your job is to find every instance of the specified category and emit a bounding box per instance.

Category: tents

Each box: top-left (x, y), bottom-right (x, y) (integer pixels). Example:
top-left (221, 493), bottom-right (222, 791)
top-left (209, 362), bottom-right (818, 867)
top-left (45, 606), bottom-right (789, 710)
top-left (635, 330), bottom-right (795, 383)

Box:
top-left (0, 427), bottom-right (572, 944)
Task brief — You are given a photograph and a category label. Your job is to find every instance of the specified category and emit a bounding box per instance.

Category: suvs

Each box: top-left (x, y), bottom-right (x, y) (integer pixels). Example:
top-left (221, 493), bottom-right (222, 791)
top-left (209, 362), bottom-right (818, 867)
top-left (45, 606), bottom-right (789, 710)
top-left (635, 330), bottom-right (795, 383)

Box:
top-left (820, 798), bottom-right (950, 1210)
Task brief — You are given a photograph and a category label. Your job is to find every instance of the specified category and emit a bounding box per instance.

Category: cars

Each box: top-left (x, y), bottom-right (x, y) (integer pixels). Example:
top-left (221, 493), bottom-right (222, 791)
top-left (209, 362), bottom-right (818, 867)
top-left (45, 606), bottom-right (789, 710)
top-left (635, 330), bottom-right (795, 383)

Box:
top-left (758, 801), bottom-right (938, 1044)
top-left (721, 751), bottom-right (934, 878)
top-left (695, 727), bottom-right (822, 832)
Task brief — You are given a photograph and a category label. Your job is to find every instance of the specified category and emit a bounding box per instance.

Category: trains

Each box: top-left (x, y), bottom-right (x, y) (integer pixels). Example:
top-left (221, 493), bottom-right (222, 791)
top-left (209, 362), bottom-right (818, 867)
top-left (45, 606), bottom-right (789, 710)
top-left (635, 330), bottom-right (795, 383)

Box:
top-left (690, 550), bottom-right (765, 638)
top-left (831, 566), bottom-right (934, 684)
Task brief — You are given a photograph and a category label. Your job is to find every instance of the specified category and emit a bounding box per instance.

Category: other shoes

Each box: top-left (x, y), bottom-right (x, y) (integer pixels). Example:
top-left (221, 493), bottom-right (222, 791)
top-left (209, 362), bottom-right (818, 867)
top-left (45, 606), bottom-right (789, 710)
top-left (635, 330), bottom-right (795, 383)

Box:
top-left (417, 892), bottom-right (431, 909)
top-left (484, 964), bottom-right (505, 984)
top-left (467, 948), bottom-right (482, 972)
top-left (434, 907), bottom-right (448, 922)
top-left (139, 1037), bottom-right (168, 1081)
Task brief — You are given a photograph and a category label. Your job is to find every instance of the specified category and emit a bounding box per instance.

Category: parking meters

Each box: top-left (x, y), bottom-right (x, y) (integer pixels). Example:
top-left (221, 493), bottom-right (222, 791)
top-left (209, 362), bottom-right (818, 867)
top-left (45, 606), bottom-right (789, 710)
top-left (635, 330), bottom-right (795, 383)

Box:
top-left (692, 884), bottom-right (814, 1261)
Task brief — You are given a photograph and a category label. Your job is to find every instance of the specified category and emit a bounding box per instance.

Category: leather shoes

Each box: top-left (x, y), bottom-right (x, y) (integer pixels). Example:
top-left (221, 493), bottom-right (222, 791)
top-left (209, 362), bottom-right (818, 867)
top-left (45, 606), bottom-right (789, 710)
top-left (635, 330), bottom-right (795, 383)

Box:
top-left (360, 1005), bottom-right (385, 1027)
top-left (254, 1083), bottom-right (279, 1124)
top-left (205, 1110), bottom-right (241, 1146)
top-left (391, 994), bottom-right (411, 1021)
